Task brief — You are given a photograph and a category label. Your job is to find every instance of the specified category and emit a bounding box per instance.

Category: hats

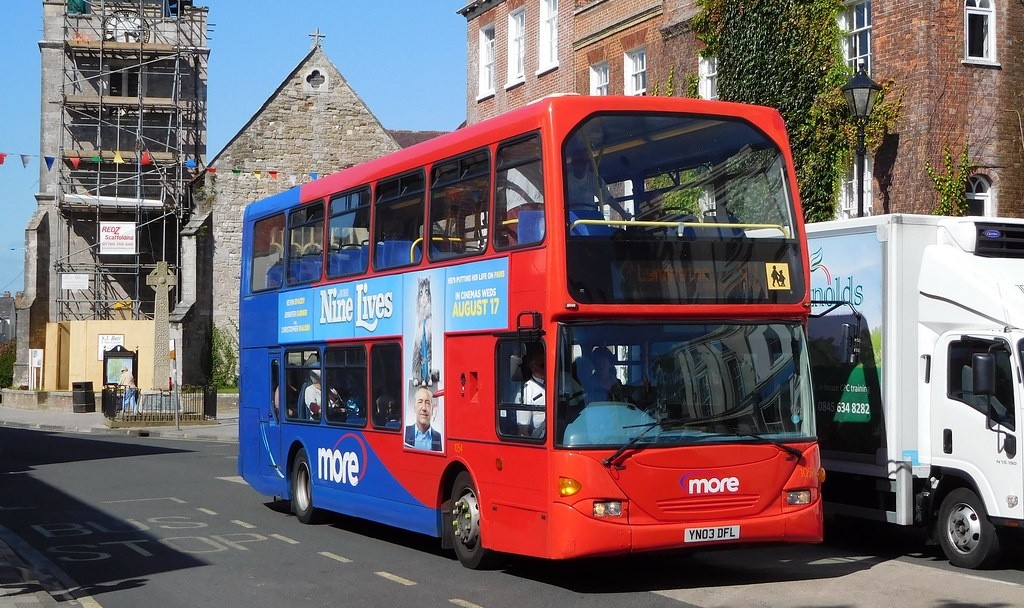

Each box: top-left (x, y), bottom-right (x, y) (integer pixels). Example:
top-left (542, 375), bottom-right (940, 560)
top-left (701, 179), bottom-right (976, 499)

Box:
top-left (121, 366), bottom-right (128, 372)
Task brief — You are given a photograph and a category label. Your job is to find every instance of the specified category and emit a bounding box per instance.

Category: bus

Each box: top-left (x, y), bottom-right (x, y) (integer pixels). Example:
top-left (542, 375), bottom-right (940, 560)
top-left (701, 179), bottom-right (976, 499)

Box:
top-left (238, 92), bottom-right (864, 571)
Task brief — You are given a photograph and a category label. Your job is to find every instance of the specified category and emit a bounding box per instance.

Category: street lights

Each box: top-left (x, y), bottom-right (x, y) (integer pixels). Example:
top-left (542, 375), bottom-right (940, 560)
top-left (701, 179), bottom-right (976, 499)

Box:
top-left (841, 59), bottom-right (884, 218)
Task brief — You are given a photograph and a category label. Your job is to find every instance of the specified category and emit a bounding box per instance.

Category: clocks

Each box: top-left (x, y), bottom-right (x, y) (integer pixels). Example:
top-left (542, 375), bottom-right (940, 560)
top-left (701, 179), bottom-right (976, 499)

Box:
top-left (104, 10), bottom-right (150, 43)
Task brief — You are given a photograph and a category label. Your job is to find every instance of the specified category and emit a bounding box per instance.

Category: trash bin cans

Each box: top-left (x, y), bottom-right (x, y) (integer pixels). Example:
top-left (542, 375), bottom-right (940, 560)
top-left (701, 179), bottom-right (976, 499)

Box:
top-left (73, 382), bottom-right (96, 413)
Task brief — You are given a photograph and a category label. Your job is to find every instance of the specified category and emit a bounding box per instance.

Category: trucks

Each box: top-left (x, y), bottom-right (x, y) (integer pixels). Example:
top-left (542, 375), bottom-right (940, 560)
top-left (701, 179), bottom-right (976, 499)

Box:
top-left (805, 213), bottom-right (1022, 569)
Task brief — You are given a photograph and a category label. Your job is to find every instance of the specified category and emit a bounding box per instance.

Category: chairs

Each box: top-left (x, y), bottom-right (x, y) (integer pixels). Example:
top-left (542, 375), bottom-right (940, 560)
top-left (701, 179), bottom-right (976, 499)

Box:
top-left (268, 206), bottom-right (749, 446)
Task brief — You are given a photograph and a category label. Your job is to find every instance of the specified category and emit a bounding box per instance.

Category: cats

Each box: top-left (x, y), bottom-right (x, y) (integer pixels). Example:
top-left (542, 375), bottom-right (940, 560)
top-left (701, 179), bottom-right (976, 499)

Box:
top-left (411, 276), bottom-right (440, 387)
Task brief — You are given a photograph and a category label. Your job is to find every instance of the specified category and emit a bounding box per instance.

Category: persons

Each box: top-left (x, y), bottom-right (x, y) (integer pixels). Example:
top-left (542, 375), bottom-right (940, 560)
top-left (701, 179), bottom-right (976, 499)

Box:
top-left (275, 362), bottom-right (401, 425)
top-left (406, 385), bottom-right (442, 451)
top-left (515, 341), bottom-right (624, 436)
top-left (118, 367), bottom-right (138, 415)
